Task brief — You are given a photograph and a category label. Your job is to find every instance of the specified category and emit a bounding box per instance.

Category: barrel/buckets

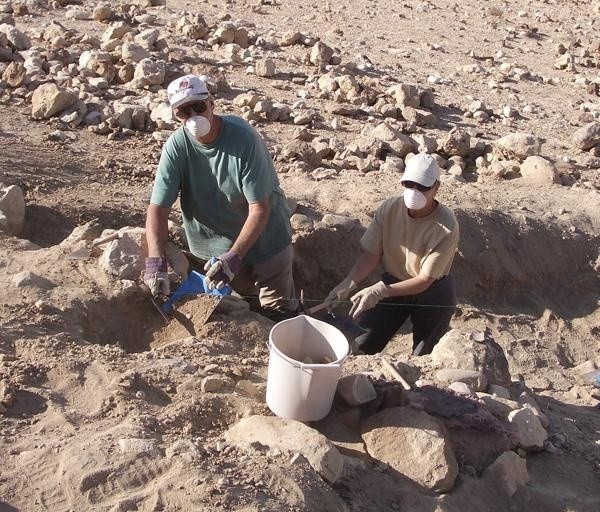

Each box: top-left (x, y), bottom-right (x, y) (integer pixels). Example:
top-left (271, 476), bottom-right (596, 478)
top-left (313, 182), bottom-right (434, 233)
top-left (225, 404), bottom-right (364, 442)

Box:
top-left (266, 315), bottom-right (352, 421)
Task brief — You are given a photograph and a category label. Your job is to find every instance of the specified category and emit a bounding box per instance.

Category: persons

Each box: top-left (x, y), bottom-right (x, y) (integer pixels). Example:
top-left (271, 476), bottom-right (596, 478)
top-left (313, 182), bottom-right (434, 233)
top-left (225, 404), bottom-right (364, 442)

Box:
top-left (141, 75), bottom-right (308, 323)
top-left (325, 152), bottom-right (461, 355)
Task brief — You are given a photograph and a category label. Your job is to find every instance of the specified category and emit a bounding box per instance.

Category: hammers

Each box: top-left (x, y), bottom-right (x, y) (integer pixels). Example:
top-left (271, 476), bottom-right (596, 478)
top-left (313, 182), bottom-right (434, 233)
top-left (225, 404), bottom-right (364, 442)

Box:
top-left (297, 289), bottom-right (332, 316)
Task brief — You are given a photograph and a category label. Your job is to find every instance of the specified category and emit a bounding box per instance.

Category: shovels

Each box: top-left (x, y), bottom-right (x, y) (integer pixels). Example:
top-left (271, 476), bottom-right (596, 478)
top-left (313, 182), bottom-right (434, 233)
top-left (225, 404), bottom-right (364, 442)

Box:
top-left (161, 256), bottom-right (231, 326)
top-left (332, 296), bottom-right (369, 341)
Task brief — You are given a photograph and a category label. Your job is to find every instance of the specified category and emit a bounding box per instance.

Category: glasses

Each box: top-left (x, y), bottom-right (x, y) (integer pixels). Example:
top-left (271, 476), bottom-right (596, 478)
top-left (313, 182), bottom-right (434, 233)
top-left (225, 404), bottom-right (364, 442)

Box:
top-left (176, 101), bottom-right (208, 119)
top-left (401, 180), bottom-right (437, 192)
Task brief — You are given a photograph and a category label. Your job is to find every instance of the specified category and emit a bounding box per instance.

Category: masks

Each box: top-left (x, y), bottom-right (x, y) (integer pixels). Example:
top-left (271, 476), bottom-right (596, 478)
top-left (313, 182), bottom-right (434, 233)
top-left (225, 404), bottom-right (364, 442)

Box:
top-left (185, 115), bottom-right (212, 139)
top-left (403, 180), bottom-right (438, 210)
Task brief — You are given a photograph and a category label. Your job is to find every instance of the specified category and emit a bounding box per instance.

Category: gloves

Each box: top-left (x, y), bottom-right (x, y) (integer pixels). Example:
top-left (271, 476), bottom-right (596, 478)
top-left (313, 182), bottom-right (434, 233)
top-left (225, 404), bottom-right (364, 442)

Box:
top-left (350, 280), bottom-right (390, 322)
top-left (204, 251), bottom-right (241, 290)
top-left (324, 278), bottom-right (358, 314)
top-left (144, 257), bottom-right (170, 296)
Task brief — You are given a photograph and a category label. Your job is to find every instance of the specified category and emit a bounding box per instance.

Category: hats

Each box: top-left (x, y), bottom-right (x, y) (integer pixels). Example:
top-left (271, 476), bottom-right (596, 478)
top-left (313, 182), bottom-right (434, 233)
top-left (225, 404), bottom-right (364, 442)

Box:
top-left (397, 153), bottom-right (441, 187)
top-left (167, 74), bottom-right (210, 109)
